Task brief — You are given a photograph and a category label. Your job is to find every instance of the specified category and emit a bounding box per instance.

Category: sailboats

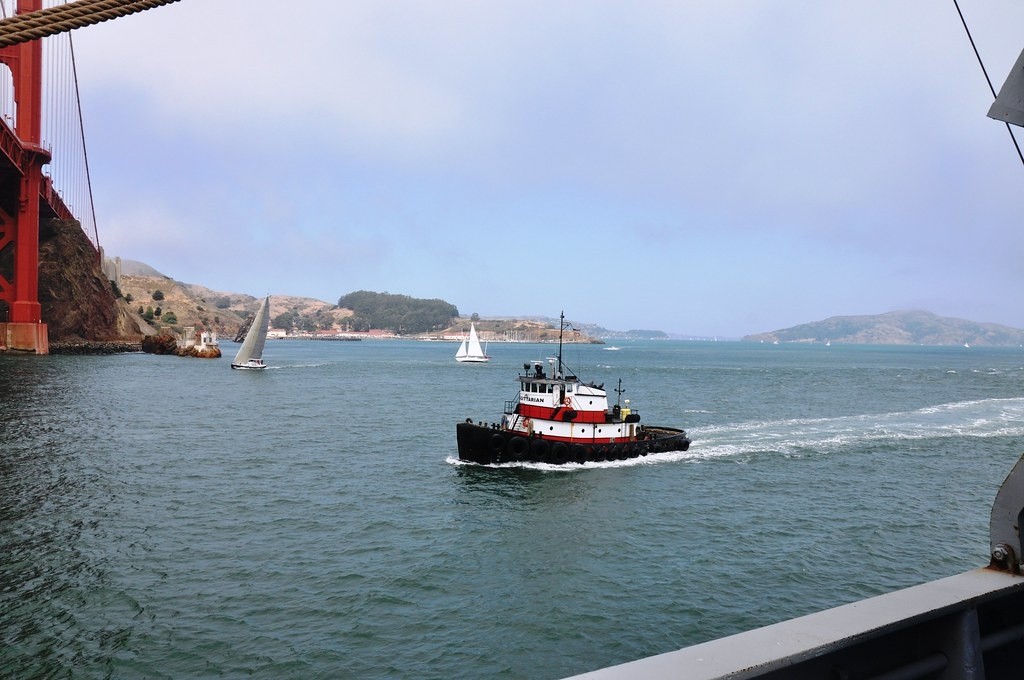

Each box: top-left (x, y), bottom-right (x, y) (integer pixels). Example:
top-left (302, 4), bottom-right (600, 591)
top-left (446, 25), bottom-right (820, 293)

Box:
top-left (454, 321), bottom-right (492, 363)
top-left (230, 296), bottom-right (274, 370)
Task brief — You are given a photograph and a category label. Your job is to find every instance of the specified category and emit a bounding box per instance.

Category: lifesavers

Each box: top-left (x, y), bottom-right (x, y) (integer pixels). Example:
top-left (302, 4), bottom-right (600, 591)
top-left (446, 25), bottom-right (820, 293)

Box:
top-left (522, 417), bottom-right (531, 428)
top-left (489, 432), bottom-right (689, 466)
top-left (564, 397), bottom-right (571, 406)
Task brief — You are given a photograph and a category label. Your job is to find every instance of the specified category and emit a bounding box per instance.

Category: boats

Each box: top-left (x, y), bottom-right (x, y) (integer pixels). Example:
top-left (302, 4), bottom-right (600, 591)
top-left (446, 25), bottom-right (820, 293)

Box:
top-left (452, 310), bottom-right (694, 468)
